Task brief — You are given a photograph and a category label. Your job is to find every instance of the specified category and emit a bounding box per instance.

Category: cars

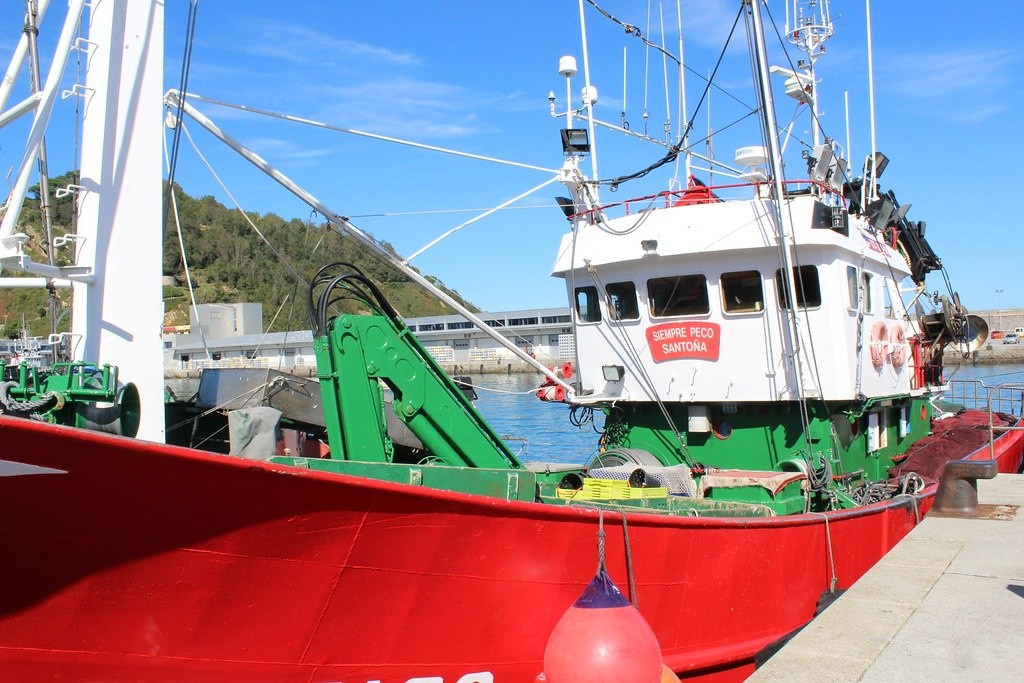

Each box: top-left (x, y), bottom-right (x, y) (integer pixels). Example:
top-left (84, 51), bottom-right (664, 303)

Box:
top-left (991, 330), bottom-right (1004, 339)
top-left (1003, 334), bottom-right (1021, 345)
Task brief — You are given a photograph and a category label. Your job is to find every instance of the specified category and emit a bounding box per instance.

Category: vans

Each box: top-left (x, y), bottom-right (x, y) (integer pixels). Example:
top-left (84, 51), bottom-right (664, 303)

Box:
top-left (1014, 327), bottom-right (1024, 338)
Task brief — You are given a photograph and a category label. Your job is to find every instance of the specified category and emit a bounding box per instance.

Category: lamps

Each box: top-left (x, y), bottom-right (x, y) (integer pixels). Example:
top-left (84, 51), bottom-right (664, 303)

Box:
top-left (555, 197), bottom-right (573, 217)
top-left (829, 157), bottom-right (847, 187)
top-left (870, 199), bottom-right (912, 235)
top-left (602, 365), bottom-right (624, 384)
top-left (804, 144), bottom-right (833, 182)
top-left (561, 129), bottom-right (590, 154)
top-left (862, 151), bottom-right (889, 178)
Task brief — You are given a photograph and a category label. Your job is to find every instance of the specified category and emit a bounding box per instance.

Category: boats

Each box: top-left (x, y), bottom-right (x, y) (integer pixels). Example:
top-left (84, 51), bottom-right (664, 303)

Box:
top-left (11, 312), bottom-right (43, 378)
top-left (0, 1), bottom-right (1024, 683)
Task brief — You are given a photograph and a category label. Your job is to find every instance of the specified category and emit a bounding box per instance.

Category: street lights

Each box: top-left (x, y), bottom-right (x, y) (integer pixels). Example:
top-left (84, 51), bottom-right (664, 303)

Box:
top-left (995, 290), bottom-right (1003, 330)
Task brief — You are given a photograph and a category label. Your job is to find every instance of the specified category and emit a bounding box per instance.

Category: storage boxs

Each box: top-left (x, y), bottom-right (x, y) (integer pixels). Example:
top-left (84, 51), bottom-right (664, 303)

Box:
top-left (555, 477), bottom-right (668, 500)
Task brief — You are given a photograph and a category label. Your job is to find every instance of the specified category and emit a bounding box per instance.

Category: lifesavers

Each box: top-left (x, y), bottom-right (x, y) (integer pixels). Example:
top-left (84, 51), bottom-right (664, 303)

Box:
top-left (890, 325), bottom-right (906, 367)
top-left (871, 320), bottom-right (888, 367)
